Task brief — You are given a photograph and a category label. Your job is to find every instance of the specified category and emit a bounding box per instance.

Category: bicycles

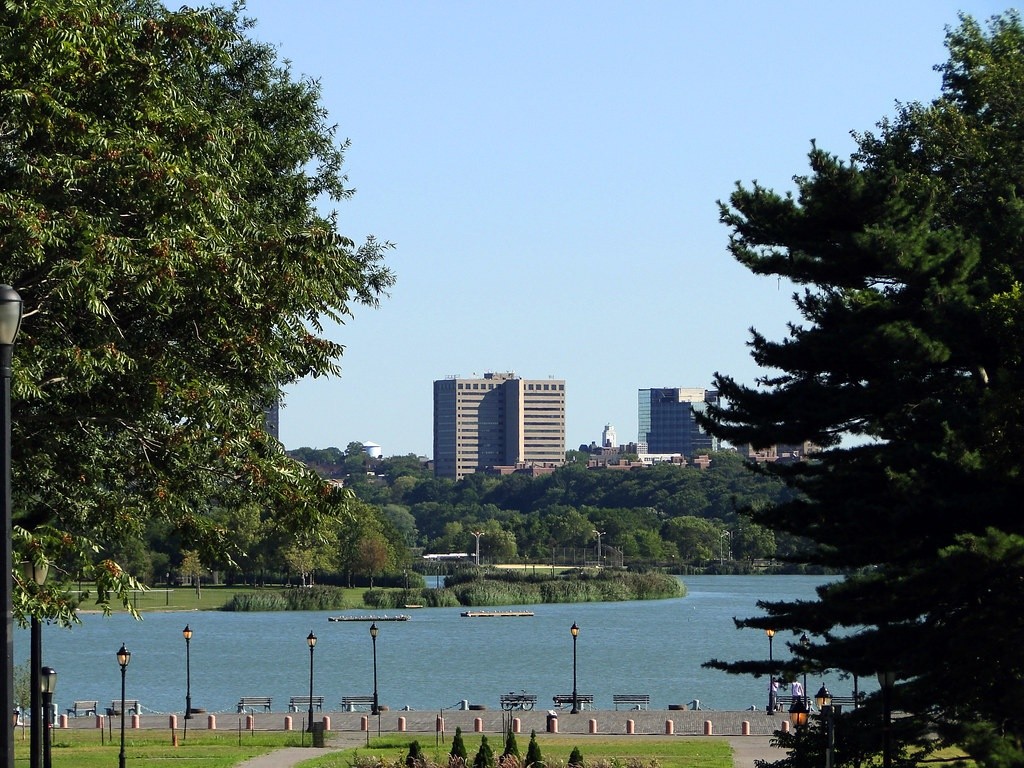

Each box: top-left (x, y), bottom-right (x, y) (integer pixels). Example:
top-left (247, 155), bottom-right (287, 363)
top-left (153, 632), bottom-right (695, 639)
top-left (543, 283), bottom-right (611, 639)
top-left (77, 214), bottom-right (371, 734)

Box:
top-left (501, 689), bottom-right (533, 710)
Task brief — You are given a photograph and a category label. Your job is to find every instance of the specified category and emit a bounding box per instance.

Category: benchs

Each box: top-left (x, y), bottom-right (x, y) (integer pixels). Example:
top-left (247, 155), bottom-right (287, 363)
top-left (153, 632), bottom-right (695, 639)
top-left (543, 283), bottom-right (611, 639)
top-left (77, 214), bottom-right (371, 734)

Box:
top-left (240, 697), bottom-right (271, 713)
top-left (556, 694), bottom-right (593, 713)
top-left (340, 695), bottom-right (374, 712)
top-left (288, 696), bottom-right (324, 713)
top-left (613, 695), bottom-right (651, 711)
top-left (832, 696), bottom-right (856, 706)
top-left (105, 700), bottom-right (138, 716)
top-left (776, 696), bottom-right (811, 713)
top-left (66, 701), bottom-right (97, 719)
top-left (501, 694), bottom-right (538, 710)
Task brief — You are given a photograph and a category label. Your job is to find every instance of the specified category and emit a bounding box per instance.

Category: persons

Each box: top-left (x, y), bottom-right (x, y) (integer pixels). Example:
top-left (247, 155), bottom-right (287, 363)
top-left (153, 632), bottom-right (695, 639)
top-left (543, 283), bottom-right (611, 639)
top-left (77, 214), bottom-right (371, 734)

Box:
top-left (788, 679), bottom-right (804, 711)
top-left (772, 677), bottom-right (779, 711)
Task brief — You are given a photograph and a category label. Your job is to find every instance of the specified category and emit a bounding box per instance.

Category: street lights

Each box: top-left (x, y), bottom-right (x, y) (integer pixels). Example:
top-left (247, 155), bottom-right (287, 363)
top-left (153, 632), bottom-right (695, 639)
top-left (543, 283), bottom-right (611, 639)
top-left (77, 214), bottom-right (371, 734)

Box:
top-left (766, 629), bottom-right (774, 714)
top-left (20, 544), bottom-right (50, 768)
top-left (799, 631), bottom-right (809, 710)
top-left (813, 681), bottom-right (834, 768)
top-left (183, 624), bottom-right (193, 719)
top-left (117, 642), bottom-right (131, 768)
top-left (570, 621), bottom-right (579, 714)
top-left (719, 528), bottom-right (742, 566)
top-left (40, 667), bottom-right (58, 768)
top-left (307, 630), bottom-right (318, 733)
top-left (470, 531), bottom-right (484, 565)
top-left (593, 530), bottom-right (607, 566)
top-left (370, 621), bottom-right (381, 715)
